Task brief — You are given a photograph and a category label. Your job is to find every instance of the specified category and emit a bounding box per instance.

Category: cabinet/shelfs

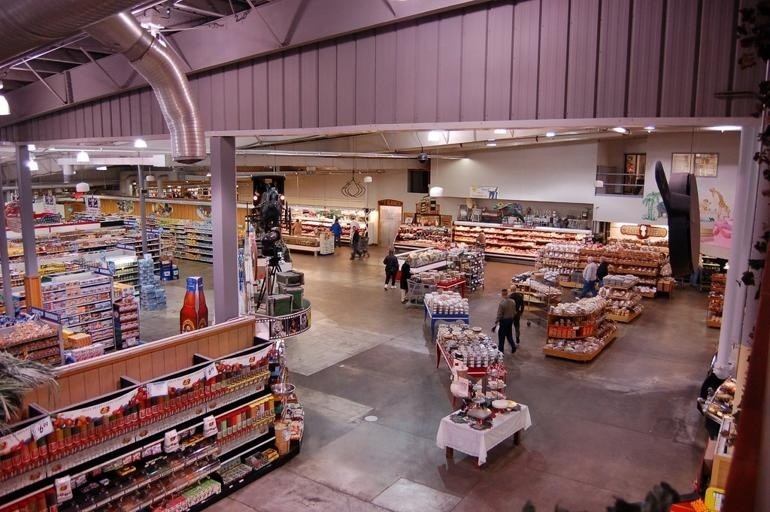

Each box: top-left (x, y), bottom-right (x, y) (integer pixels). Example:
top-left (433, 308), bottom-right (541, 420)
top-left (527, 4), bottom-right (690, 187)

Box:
top-left (0, 211), bottom-right (311, 512)
top-left (396, 216), bottom-right (727, 360)
top-left (280, 212), bottom-right (378, 256)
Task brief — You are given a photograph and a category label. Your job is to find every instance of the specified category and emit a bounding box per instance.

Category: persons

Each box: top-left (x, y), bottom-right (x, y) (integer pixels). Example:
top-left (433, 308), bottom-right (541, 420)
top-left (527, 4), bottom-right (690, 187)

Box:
top-left (576, 256), bottom-right (597, 299)
top-left (382, 248), bottom-right (399, 291)
top-left (347, 216), bottom-right (364, 260)
top-left (400, 254), bottom-right (413, 303)
top-left (292, 218), bottom-right (302, 235)
top-left (594, 257), bottom-right (609, 286)
top-left (491, 289), bottom-right (517, 355)
top-left (329, 218), bottom-right (343, 248)
top-left (508, 286), bottom-right (524, 343)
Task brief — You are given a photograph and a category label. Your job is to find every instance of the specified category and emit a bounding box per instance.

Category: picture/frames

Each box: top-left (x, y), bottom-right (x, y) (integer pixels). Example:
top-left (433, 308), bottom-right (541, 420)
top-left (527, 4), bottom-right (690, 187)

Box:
top-left (695, 154), bottom-right (719, 176)
top-left (671, 152), bottom-right (694, 175)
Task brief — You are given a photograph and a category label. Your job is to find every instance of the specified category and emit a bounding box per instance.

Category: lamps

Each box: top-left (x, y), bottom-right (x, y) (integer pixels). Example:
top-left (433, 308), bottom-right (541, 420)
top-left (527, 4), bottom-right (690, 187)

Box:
top-left (364, 171), bottom-right (375, 185)
top-left (428, 145), bottom-right (444, 198)
top-left (0, 80), bottom-right (150, 173)
top-left (594, 134), bottom-right (605, 188)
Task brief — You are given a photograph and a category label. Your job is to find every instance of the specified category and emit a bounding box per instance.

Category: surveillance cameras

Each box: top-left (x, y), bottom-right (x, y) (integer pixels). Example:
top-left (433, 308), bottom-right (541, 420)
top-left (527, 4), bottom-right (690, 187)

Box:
top-left (417, 152), bottom-right (429, 164)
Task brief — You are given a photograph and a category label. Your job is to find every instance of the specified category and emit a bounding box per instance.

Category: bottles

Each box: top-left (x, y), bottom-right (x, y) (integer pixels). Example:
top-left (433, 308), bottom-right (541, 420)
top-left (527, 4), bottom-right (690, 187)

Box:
top-left (180, 276), bottom-right (197, 333)
top-left (196, 278), bottom-right (207, 328)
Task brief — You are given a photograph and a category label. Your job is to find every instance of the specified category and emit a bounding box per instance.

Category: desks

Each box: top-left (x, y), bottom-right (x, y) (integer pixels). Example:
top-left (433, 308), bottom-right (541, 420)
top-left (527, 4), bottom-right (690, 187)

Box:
top-left (436, 399), bottom-right (532, 469)
top-left (435, 340), bottom-right (508, 407)
